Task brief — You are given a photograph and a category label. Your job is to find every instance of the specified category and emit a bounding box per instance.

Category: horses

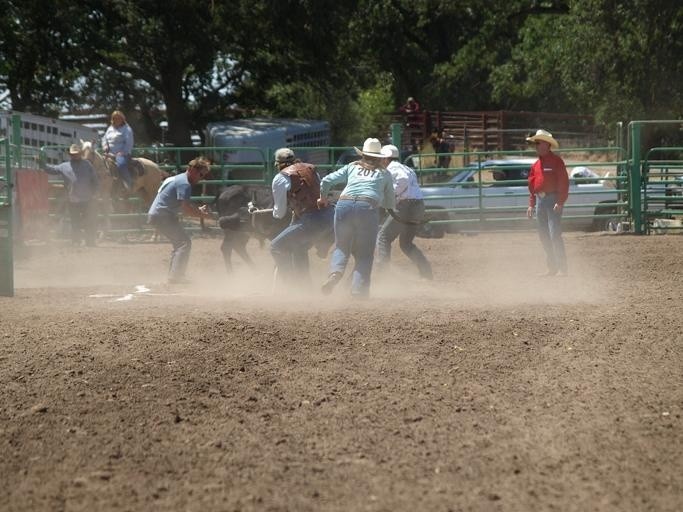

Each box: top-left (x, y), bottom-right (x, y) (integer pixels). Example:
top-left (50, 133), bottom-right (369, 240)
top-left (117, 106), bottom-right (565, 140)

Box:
top-left (78, 136), bottom-right (167, 243)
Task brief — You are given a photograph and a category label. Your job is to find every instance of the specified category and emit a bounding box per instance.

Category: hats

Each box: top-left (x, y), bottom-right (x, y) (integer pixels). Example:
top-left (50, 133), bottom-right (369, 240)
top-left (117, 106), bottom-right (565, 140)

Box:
top-left (274, 148), bottom-right (294, 166)
top-left (525, 130), bottom-right (567, 147)
top-left (353, 137), bottom-right (392, 158)
top-left (381, 145), bottom-right (400, 158)
top-left (488, 169), bottom-right (506, 177)
top-left (69, 144), bottom-right (83, 156)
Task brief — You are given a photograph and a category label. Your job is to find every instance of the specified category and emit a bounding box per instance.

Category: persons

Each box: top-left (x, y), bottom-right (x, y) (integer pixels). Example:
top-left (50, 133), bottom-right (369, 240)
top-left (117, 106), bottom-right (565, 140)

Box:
top-left (526, 127), bottom-right (573, 280)
top-left (493, 169), bottom-right (506, 181)
top-left (34, 98), bottom-right (455, 300)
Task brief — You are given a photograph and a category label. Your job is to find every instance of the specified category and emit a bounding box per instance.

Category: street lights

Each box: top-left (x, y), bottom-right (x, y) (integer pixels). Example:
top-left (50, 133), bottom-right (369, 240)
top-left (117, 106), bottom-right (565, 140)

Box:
top-left (159, 120), bottom-right (168, 146)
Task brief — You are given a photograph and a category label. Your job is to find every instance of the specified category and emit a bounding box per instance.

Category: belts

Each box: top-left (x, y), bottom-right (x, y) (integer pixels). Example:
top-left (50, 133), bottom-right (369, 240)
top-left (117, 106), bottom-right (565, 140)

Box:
top-left (340, 196), bottom-right (378, 210)
top-left (538, 192), bottom-right (546, 197)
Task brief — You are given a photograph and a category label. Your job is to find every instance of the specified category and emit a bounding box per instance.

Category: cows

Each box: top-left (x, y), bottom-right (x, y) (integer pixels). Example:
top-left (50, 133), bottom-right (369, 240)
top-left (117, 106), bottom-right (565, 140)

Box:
top-left (200, 183), bottom-right (335, 276)
top-left (219, 203), bottom-right (433, 296)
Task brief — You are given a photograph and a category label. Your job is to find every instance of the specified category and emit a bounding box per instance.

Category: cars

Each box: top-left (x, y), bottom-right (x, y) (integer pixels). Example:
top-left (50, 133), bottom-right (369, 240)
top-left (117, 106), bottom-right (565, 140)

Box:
top-left (665, 176), bottom-right (683, 223)
top-left (413, 158), bottom-right (629, 239)
